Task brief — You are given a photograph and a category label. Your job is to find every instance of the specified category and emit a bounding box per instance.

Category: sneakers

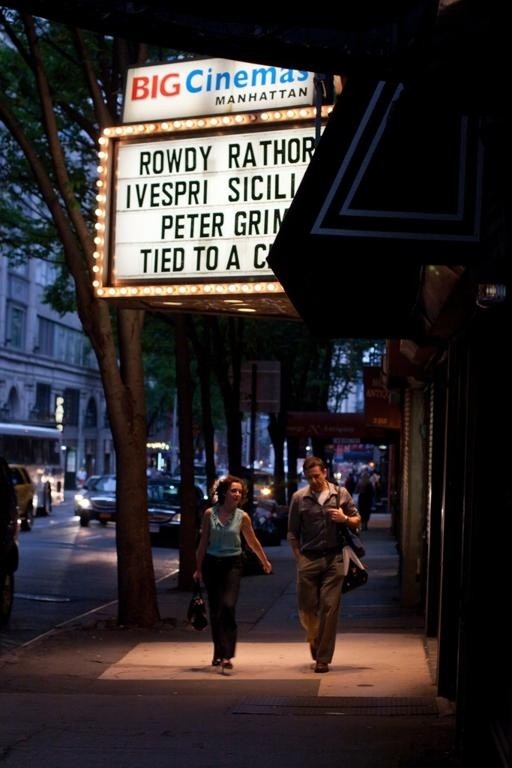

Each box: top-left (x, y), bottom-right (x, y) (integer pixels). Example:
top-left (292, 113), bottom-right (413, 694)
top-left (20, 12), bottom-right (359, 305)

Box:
top-left (211, 660), bottom-right (233, 669)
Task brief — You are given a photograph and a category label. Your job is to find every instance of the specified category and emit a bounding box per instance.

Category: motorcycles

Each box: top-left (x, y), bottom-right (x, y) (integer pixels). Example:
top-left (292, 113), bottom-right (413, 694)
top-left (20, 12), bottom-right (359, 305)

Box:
top-left (254, 498), bottom-right (286, 546)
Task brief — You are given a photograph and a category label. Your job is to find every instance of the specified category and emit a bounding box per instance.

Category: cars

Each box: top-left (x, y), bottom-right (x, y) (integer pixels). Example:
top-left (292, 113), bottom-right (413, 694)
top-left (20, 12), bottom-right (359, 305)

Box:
top-left (75, 469), bottom-right (274, 544)
top-left (0, 456), bottom-right (38, 626)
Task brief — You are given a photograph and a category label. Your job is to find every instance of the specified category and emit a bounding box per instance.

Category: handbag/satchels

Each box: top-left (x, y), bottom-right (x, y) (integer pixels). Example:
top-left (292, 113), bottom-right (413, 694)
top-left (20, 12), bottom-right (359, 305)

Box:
top-left (342, 544), bottom-right (368, 593)
top-left (187, 579), bottom-right (209, 631)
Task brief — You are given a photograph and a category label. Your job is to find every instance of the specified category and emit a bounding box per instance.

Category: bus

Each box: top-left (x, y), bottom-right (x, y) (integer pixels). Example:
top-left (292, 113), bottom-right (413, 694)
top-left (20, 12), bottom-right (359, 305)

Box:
top-left (0, 422), bottom-right (65, 516)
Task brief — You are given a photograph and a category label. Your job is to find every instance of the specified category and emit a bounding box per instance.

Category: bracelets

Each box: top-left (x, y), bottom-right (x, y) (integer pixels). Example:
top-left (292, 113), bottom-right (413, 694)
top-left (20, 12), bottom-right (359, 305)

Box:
top-left (344, 514), bottom-right (350, 524)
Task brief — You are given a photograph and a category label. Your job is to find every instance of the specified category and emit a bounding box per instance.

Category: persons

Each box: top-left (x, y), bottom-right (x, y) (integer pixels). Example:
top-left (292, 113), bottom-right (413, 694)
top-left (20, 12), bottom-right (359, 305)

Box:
top-left (285, 456), bottom-right (364, 675)
top-left (192, 476), bottom-right (274, 674)
top-left (357, 473), bottom-right (373, 531)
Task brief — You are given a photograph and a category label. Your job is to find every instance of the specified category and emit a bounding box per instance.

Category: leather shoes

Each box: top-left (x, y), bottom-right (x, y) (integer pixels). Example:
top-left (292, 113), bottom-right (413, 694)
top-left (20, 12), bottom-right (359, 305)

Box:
top-left (314, 660), bottom-right (329, 672)
